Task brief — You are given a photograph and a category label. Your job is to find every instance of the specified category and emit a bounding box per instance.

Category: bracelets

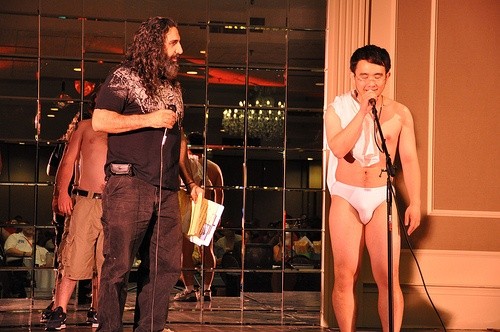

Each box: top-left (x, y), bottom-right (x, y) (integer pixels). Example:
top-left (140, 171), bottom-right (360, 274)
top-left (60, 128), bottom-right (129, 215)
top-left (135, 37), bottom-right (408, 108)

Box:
top-left (185, 181), bottom-right (194, 187)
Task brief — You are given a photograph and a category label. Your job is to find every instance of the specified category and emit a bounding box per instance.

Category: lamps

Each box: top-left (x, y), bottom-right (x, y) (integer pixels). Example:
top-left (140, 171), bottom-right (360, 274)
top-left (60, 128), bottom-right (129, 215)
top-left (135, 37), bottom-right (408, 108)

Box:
top-left (222, 86), bottom-right (285, 139)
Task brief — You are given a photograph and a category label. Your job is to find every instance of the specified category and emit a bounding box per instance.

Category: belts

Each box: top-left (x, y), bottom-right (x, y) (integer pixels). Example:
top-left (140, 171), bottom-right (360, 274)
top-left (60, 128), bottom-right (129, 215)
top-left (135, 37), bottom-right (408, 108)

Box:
top-left (70, 188), bottom-right (103, 199)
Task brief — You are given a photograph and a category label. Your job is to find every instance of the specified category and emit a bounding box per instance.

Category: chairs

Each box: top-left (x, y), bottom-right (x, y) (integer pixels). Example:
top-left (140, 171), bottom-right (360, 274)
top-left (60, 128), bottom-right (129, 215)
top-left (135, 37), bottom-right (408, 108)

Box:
top-left (0, 227), bottom-right (32, 297)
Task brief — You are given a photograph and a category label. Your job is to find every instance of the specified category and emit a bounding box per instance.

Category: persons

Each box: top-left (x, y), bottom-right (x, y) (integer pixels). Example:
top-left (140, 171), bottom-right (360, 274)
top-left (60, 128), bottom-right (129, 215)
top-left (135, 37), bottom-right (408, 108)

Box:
top-left (172, 131), bottom-right (224, 302)
top-left (45, 82), bottom-right (108, 331)
top-left (40, 110), bottom-right (94, 324)
top-left (0, 215), bottom-right (299, 267)
top-left (92, 18), bottom-right (205, 332)
top-left (323, 44), bottom-right (421, 332)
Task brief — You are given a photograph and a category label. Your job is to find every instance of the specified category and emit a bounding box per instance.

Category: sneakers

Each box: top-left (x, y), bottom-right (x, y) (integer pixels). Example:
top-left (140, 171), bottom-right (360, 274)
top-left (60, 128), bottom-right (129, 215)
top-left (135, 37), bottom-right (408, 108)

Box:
top-left (174, 288), bottom-right (198, 302)
top-left (91, 314), bottom-right (101, 327)
top-left (198, 290), bottom-right (213, 302)
top-left (40, 301), bottom-right (54, 322)
top-left (85, 307), bottom-right (96, 323)
top-left (45, 306), bottom-right (67, 330)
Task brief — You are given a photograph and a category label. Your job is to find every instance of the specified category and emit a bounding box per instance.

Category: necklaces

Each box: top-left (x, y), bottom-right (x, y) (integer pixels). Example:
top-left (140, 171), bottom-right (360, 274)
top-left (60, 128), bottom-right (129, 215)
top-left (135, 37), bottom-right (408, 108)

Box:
top-left (356, 94), bottom-right (383, 133)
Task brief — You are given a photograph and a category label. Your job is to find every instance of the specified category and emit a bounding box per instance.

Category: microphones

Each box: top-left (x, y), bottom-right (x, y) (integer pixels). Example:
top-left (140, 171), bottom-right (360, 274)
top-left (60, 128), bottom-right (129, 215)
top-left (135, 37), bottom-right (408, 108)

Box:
top-left (367, 89), bottom-right (377, 106)
top-left (162, 105), bottom-right (177, 146)
